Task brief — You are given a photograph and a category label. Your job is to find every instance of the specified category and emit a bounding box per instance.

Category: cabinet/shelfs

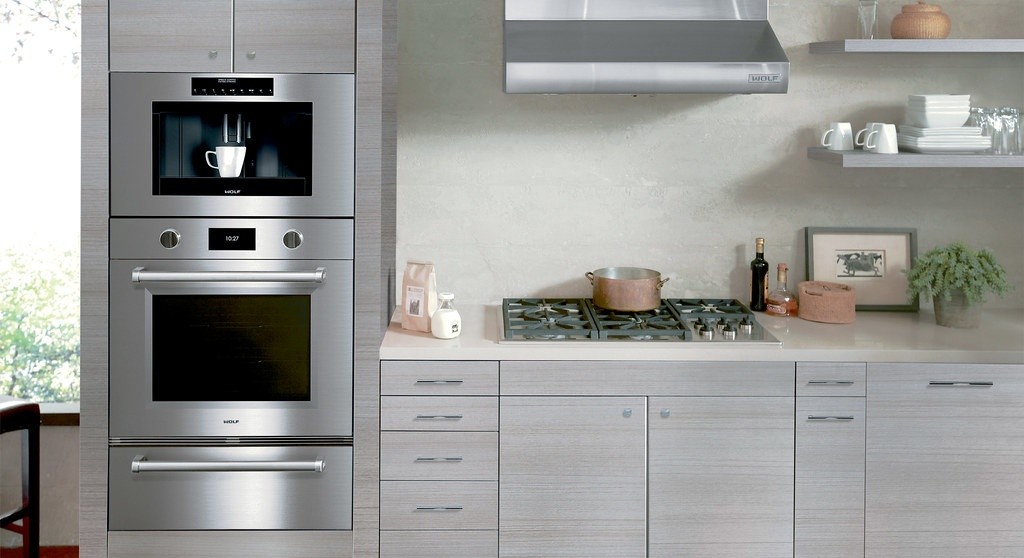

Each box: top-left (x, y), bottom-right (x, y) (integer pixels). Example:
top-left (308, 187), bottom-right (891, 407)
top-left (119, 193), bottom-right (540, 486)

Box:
top-left (378, 360), bottom-right (498, 557)
top-left (108, 0), bottom-right (356, 74)
top-left (807, 38), bottom-right (1024, 171)
top-left (795, 362), bottom-right (865, 557)
top-left (869, 363), bottom-right (1024, 557)
top-left (498, 359), bottom-right (797, 558)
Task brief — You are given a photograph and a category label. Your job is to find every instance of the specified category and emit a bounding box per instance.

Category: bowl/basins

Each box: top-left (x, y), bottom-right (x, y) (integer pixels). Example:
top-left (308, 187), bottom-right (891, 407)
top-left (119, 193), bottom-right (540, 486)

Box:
top-left (908, 95), bottom-right (970, 128)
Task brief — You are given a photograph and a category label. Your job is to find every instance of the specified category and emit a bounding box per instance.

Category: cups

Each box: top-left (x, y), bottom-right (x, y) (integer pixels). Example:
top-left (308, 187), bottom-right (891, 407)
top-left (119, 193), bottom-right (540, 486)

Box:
top-left (205, 145), bottom-right (246, 177)
top-left (855, 122), bottom-right (886, 150)
top-left (821, 122), bottom-right (854, 151)
top-left (856, 0), bottom-right (879, 39)
top-left (866, 123), bottom-right (898, 154)
top-left (969, 107), bottom-right (1024, 156)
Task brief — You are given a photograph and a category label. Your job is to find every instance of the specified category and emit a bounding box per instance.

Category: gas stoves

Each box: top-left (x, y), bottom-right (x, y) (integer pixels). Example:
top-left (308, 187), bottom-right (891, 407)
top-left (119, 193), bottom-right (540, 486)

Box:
top-left (502, 296), bottom-right (782, 348)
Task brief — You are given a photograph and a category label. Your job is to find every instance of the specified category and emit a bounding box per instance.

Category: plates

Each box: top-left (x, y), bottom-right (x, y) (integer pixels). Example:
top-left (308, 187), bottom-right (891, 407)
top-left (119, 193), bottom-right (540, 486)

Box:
top-left (897, 124), bottom-right (992, 154)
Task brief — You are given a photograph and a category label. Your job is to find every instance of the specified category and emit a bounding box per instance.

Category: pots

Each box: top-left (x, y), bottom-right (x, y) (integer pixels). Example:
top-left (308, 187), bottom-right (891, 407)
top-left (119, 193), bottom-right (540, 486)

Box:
top-left (585, 267), bottom-right (670, 313)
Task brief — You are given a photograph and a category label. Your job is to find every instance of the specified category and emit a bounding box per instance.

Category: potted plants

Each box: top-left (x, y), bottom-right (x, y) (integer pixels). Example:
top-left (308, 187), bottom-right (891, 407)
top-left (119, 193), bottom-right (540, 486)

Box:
top-left (909, 243), bottom-right (1008, 327)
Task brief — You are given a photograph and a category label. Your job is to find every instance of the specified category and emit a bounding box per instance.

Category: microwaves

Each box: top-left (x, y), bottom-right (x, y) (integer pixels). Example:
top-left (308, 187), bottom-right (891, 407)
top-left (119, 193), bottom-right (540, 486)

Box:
top-left (107, 71), bottom-right (357, 218)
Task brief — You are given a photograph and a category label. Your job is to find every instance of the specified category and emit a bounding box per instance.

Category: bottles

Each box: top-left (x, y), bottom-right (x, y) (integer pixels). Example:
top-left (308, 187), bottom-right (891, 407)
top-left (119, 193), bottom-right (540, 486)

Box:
top-left (765, 263), bottom-right (799, 315)
top-left (750, 238), bottom-right (769, 311)
top-left (431, 293), bottom-right (462, 339)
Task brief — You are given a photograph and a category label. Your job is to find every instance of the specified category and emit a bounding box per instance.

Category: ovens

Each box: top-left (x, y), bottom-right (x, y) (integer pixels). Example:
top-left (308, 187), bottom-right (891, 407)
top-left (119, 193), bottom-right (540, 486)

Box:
top-left (107, 217), bottom-right (354, 531)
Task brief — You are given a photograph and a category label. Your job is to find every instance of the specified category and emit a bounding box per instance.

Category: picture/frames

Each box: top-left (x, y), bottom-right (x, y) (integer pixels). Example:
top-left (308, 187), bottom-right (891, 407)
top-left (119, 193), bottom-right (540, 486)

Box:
top-left (804, 226), bottom-right (921, 316)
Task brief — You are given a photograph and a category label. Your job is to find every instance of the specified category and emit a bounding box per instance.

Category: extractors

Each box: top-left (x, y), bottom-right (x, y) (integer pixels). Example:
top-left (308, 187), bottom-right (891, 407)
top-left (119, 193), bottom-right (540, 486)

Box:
top-left (504, 0), bottom-right (790, 96)
